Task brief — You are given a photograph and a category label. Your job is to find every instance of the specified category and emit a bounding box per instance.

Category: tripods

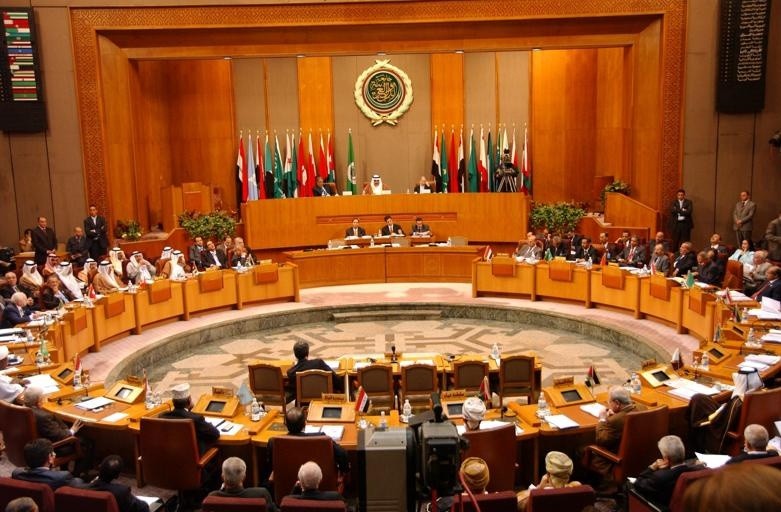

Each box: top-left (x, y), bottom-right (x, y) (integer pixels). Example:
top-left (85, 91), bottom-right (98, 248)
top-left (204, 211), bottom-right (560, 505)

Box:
top-left (498, 175), bottom-right (516, 192)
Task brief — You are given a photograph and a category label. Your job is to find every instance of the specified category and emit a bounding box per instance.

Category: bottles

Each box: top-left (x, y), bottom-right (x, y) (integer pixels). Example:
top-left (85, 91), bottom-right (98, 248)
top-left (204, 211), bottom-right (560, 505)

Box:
top-left (381, 410), bottom-right (387, 430)
top-left (746, 326), bottom-right (757, 346)
top-left (128, 279), bottom-right (135, 291)
top-left (237, 260), bottom-right (242, 272)
top-left (623, 370), bottom-right (642, 397)
top-left (403, 398), bottom-right (412, 422)
top-left (37, 349), bottom-right (49, 366)
top-left (701, 351), bottom-right (710, 371)
top-left (82, 292), bottom-right (94, 307)
top-left (251, 397), bottom-right (268, 420)
top-left (74, 370), bottom-right (83, 386)
top-left (587, 256), bottom-right (594, 270)
top-left (642, 263), bottom-right (649, 275)
top-left (146, 391), bottom-right (162, 410)
top-left (58, 297), bottom-right (65, 315)
top-left (27, 328), bottom-right (33, 345)
top-left (741, 306), bottom-right (748, 324)
top-left (531, 251), bottom-right (536, 263)
top-left (537, 392), bottom-right (550, 417)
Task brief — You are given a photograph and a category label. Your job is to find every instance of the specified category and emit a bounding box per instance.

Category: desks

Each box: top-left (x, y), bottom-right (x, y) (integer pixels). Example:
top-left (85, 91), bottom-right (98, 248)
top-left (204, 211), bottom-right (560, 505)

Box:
top-left (239, 192), bottom-right (531, 252)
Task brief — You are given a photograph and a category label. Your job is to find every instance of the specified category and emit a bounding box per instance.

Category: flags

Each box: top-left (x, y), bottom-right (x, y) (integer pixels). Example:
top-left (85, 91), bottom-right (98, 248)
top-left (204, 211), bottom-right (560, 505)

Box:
top-left (236, 132), bottom-right (356, 210)
top-left (431, 129), bottom-right (531, 197)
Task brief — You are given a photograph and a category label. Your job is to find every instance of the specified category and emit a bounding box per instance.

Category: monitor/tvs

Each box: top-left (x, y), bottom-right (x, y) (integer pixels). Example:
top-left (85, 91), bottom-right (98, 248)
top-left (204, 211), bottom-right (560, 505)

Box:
top-left (322, 407), bottom-right (341, 418)
top-left (58, 368), bottom-right (73, 380)
top-left (561, 390), bottom-right (582, 402)
top-left (733, 326), bottom-right (743, 335)
top-left (447, 403), bottom-right (463, 415)
top-left (205, 400), bottom-right (226, 413)
top-left (652, 371), bottom-right (670, 382)
top-left (115, 387), bottom-right (134, 398)
top-left (709, 347), bottom-right (724, 359)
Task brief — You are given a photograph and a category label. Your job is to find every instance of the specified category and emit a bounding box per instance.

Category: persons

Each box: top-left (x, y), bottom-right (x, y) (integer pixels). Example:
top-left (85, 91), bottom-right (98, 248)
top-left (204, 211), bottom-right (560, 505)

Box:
top-left (412, 218), bottom-right (430, 236)
top-left (413, 176), bottom-right (433, 194)
top-left (345, 217), bottom-right (366, 238)
top-left (732, 191), bottom-right (757, 251)
top-left (517, 229), bottom-right (780, 303)
top-left (83, 204), bottom-right (110, 261)
top-left (671, 189), bottom-right (695, 253)
top-left (31, 216), bottom-right (58, 268)
top-left (1, 230), bottom-right (274, 511)
top-left (431, 367), bottom-right (780, 510)
top-left (765, 216), bottom-right (781, 261)
top-left (267, 341), bottom-right (360, 512)
top-left (362, 175), bottom-right (393, 195)
top-left (381, 216), bottom-right (406, 237)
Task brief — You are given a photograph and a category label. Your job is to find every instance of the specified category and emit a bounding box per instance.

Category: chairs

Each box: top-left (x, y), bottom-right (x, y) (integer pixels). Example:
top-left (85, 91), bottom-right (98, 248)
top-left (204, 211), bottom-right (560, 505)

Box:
top-left (416, 182), bottom-right (435, 194)
top-left (363, 183), bottom-right (370, 189)
top-left (324, 183), bottom-right (337, 195)
